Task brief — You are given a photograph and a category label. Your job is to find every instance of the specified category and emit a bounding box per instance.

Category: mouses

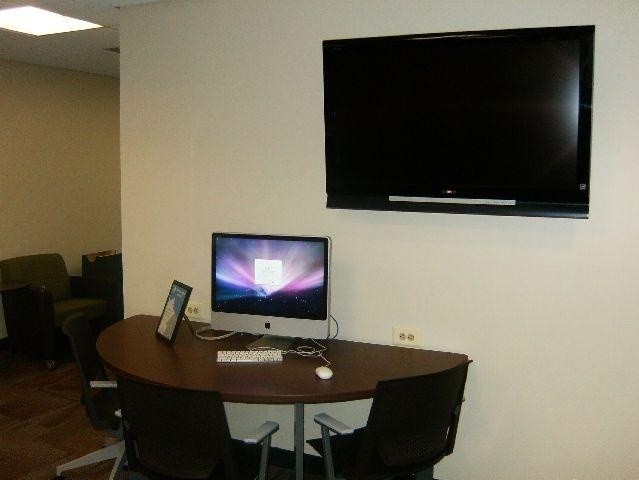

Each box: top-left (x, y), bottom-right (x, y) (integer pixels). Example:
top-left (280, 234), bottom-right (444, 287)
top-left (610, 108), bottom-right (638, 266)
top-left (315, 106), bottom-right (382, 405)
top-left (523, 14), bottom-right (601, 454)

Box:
top-left (315, 366), bottom-right (333, 380)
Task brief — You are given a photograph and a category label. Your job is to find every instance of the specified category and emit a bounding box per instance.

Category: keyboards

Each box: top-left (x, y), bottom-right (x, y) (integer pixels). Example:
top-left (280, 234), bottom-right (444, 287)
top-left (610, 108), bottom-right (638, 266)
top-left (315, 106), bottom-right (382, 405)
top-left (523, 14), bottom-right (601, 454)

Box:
top-left (216, 350), bottom-right (283, 364)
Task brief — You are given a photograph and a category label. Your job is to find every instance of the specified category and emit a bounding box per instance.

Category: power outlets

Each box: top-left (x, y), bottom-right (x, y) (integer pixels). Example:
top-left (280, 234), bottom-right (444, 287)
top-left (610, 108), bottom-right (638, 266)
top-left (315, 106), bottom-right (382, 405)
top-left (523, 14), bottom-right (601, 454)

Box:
top-left (183, 303), bottom-right (205, 319)
top-left (390, 325), bottom-right (423, 349)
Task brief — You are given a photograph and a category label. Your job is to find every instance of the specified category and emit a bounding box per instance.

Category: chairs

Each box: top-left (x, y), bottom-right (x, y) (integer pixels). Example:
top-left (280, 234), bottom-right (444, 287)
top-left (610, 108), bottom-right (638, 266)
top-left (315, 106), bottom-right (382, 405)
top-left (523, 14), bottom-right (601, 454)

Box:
top-left (306, 360), bottom-right (473, 479)
top-left (61, 312), bottom-right (128, 480)
top-left (128, 381), bottom-right (280, 480)
top-left (0, 253), bottom-right (122, 355)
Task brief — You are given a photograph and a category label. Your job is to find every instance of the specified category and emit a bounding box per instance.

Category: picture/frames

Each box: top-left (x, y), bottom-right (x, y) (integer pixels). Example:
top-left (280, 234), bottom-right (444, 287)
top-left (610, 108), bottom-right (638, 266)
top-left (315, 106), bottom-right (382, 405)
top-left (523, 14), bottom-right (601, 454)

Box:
top-left (155, 279), bottom-right (193, 346)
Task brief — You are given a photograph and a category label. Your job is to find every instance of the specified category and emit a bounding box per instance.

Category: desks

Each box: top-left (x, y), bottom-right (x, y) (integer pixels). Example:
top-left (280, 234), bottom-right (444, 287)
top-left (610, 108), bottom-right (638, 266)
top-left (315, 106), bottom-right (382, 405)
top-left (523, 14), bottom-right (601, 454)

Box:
top-left (95, 314), bottom-right (468, 405)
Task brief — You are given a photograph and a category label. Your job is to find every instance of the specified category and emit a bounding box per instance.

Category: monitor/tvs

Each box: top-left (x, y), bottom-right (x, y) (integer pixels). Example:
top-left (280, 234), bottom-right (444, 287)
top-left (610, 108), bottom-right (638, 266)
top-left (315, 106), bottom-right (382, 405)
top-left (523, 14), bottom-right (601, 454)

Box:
top-left (322, 24), bottom-right (596, 218)
top-left (211, 232), bottom-right (330, 350)
